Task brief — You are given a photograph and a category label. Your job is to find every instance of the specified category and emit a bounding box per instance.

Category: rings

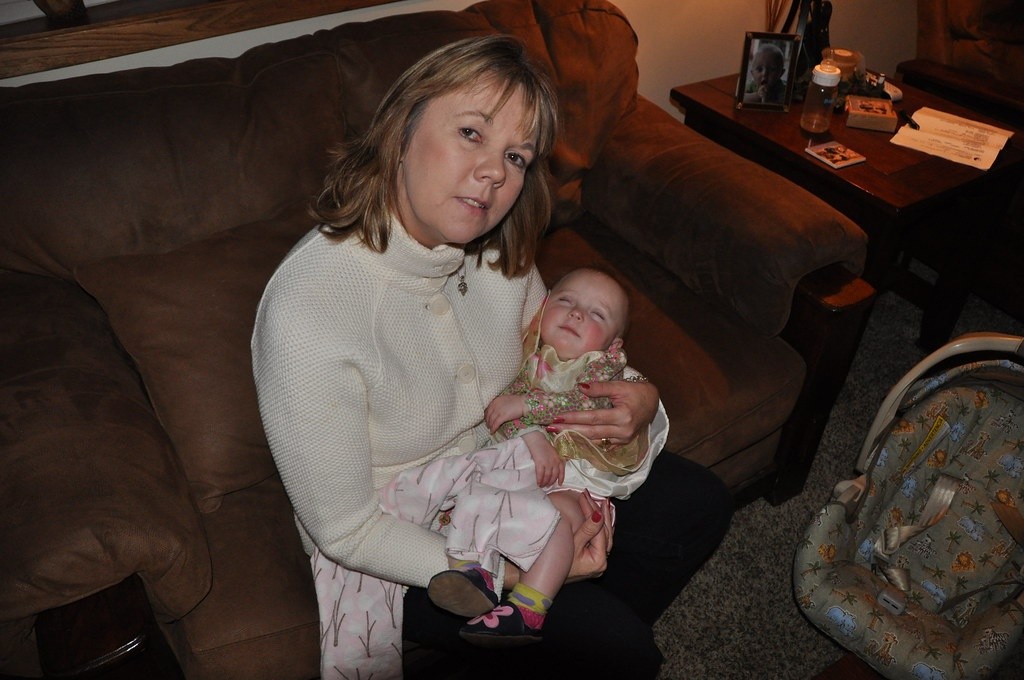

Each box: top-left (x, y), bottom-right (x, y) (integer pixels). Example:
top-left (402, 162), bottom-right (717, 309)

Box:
top-left (601, 438), bottom-right (611, 452)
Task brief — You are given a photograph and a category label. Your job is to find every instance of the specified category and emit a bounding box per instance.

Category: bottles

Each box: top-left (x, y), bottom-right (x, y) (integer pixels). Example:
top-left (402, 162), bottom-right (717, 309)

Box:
top-left (821, 48), bottom-right (862, 113)
top-left (799, 49), bottom-right (842, 134)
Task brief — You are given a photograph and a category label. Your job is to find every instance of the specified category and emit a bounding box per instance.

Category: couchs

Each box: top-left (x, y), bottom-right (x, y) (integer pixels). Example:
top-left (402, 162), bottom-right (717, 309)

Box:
top-left (0, 0), bottom-right (876, 679)
top-left (896, 0), bottom-right (1024, 111)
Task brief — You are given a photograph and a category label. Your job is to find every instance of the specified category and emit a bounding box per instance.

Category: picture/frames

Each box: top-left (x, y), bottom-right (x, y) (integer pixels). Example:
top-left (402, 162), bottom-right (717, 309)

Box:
top-left (734, 31), bottom-right (801, 113)
top-left (0, 0), bottom-right (406, 79)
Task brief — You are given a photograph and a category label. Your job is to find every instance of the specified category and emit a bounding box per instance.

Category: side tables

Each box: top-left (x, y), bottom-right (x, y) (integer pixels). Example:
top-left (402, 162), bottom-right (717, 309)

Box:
top-left (670, 68), bottom-right (1024, 350)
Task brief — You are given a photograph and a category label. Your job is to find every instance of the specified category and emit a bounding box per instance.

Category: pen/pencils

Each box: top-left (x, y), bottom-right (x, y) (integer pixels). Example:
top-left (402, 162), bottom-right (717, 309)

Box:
top-left (899, 108), bottom-right (920, 130)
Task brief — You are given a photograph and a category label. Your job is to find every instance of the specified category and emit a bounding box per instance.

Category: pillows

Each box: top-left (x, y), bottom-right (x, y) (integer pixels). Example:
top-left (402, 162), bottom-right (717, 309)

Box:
top-left (72, 195), bottom-right (324, 504)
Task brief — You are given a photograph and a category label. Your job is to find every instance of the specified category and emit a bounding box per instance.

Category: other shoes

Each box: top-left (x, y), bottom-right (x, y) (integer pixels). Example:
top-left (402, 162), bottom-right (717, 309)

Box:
top-left (458, 600), bottom-right (545, 639)
top-left (427, 568), bottom-right (501, 617)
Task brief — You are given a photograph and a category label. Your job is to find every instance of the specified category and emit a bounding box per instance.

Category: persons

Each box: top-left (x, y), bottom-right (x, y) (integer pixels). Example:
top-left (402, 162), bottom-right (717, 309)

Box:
top-left (249, 32), bottom-right (732, 680)
top-left (427, 265), bottom-right (628, 645)
top-left (744, 44), bottom-right (785, 103)
